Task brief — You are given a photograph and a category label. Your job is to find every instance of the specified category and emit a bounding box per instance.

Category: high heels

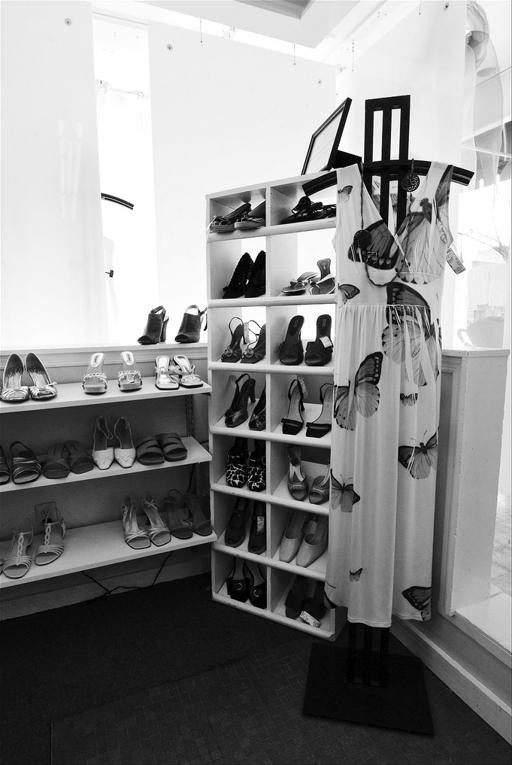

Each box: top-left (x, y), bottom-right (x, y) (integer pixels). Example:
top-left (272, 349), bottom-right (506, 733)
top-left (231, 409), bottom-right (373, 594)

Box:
top-left (278, 314), bottom-right (305, 367)
top-left (119, 491), bottom-right (150, 549)
top-left (116, 350), bottom-right (143, 392)
top-left (249, 377), bottom-right (266, 431)
top-left (283, 444), bottom-right (308, 505)
top-left (218, 315), bottom-right (246, 364)
top-left (177, 489), bottom-right (213, 538)
top-left (245, 500), bottom-right (266, 554)
top-left (309, 468), bottom-right (329, 504)
top-left (1, 352), bottom-right (28, 403)
top-left (221, 436), bottom-right (249, 492)
top-left (113, 415), bottom-right (137, 469)
top-left (296, 511), bottom-right (328, 568)
top-left (163, 485), bottom-right (191, 541)
top-left (306, 380), bottom-right (332, 439)
top-left (207, 198), bottom-right (253, 234)
top-left (154, 350), bottom-right (180, 391)
top-left (280, 376), bottom-right (306, 436)
top-left (305, 314), bottom-right (334, 366)
top-left (140, 494), bottom-right (173, 547)
top-left (281, 270), bottom-right (316, 296)
top-left (21, 353), bottom-right (58, 400)
top-left (137, 303), bottom-right (169, 345)
top-left (171, 353), bottom-right (205, 389)
top-left (242, 557), bottom-right (266, 608)
top-left (239, 317), bottom-right (266, 367)
top-left (227, 556), bottom-right (248, 609)
top-left (80, 351), bottom-right (108, 396)
top-left (239, 197), bottom-right (270, 233)
top-left (224, 371), bottom-right (256, 431)
top-left (221, 250), bottom-right (253, 298)
top-left (244, 249), bottom-right (266, 298)
top-left (246, 437), bottom-right (265, 492)
top-left (175, 307), bottom-right (202, 343)
top-left (223, 497), bottom-right (252, 549)
top-left (277, 508), bottom-right (309, 562)
top-left (90, 413), bottom-right (114, 471)
top-left (308, 257), bottom-right (337, 296)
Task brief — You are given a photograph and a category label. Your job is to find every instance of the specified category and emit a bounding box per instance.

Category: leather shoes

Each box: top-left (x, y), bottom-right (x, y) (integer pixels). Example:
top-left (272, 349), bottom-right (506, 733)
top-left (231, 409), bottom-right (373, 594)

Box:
top-left (286, 201), bottom-right (322, 224)
top-left (296, 202), bottom-right (337, 220)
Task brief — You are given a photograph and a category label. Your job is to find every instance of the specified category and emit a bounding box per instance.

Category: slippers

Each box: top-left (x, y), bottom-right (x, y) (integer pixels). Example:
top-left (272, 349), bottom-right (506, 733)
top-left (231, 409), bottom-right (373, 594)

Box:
top-left (303, 579), bottom-right (330, 624)
top-left (134, 430), bottom-right (165, 466)
top-left (284, 574), bottom-right (307, 624)
top-left (155, 429), bottom-right (188, 462)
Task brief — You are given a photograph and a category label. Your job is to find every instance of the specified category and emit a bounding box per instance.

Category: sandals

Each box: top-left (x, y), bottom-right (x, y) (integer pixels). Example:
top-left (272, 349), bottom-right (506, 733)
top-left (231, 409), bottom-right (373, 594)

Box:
top-left (11, 439), bottom-right (41, 486)
top-left (63, 439), bottom-right (95, 474)
top-left (34, 508), bottom-right (68, 569)
top-left (1, 512), bottom-right (36, 579)
top-left (41, 437), bottom-right (69, 480)
top-left (0, 445), bottom-right (10, 485)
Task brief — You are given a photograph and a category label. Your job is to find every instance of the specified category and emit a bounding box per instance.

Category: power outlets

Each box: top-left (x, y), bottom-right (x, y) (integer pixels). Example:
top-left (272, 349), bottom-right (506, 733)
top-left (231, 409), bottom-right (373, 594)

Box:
top-left (34, 502), bottom-right (58, 533)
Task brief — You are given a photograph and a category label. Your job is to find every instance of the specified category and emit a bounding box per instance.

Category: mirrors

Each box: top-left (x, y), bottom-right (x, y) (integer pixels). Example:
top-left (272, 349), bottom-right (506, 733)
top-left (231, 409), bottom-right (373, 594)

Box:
top-left (300, 97), bottom-right (362, 173)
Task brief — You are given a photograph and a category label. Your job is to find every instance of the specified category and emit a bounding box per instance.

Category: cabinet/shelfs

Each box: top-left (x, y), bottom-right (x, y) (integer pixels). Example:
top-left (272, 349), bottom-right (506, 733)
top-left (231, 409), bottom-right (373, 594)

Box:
top-left (206, 169), bottom-right (341, 641)
top-left (1, 376), bottom-right (217, 620)
